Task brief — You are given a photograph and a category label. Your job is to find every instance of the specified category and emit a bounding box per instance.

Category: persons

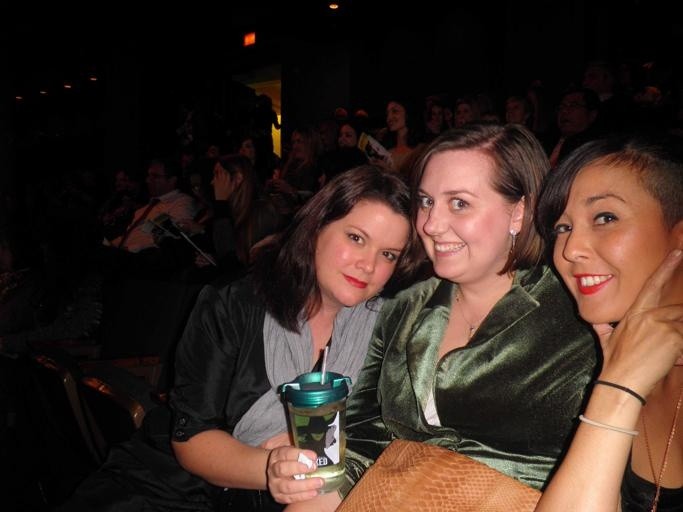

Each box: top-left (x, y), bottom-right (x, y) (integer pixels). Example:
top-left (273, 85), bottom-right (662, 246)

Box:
top-left (286, 114), bottom-right (603, 512)
top-left (68, 165), bottom-right (432, 511)
top-left (533, 117), bottom-right (682, 511)
top-left (0, 53), bottom-right (682, 431)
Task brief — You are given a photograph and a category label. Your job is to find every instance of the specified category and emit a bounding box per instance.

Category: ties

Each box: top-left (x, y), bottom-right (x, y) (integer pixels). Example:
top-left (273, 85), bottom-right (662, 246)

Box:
top-left (118, 198), bottom-right (160, 247)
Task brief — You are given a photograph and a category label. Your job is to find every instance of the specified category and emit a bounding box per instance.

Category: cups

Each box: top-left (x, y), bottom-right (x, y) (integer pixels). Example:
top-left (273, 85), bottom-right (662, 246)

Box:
top-left (281, 372), bottom-right (353, 494)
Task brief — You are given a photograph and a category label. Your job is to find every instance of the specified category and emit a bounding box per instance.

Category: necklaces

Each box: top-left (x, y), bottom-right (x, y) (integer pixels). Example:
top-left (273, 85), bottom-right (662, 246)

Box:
top-left (640, 393), bottom-right (681, 510)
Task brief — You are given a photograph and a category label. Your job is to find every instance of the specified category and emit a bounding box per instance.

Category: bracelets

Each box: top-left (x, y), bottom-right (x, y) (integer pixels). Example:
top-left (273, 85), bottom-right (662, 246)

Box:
top-left (578, 413), bottom-right (638, 437)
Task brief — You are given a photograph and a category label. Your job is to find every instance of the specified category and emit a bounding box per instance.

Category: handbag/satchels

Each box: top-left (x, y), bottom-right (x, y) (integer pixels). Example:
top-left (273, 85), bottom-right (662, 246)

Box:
top-left (337, 438), bottom-right (542, 512)
